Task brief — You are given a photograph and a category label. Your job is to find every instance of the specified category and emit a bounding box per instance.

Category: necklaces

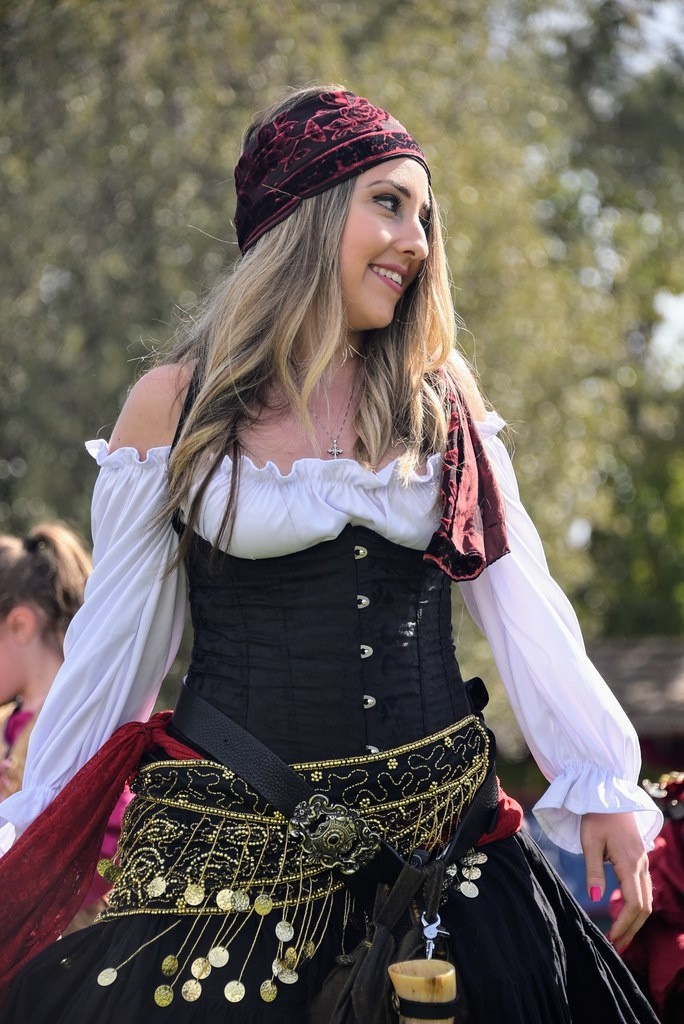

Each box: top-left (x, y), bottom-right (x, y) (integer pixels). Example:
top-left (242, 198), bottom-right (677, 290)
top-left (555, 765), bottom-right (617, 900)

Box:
top-left (279, 329), bottom-right (365, 459)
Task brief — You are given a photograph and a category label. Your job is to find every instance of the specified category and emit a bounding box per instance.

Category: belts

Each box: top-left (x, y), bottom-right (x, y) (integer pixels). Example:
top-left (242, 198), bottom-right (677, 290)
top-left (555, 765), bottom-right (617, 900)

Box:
top-left (172, 675), bottom-right (499, 920)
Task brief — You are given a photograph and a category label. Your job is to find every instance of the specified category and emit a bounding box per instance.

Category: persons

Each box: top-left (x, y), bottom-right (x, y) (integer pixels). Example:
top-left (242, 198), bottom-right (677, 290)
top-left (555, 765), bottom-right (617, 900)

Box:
top-left (0, 522), bottom-right (148, 943)
top-left (608, 771), bottom-right (683, 1024)
top-left (0, 88), bottom-right (663, 1024)
